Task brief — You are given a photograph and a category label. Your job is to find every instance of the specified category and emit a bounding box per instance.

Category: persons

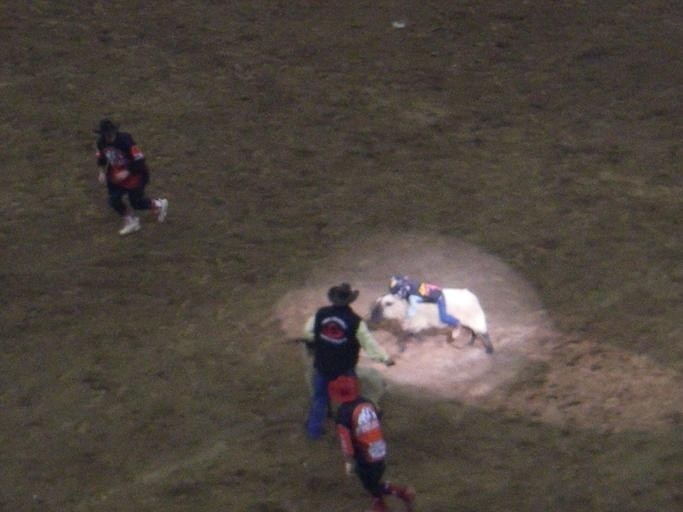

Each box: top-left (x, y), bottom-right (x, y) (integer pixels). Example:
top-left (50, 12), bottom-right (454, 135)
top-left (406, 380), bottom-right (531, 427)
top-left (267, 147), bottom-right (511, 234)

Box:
top-left (303, 282), bottom-right (394, 441)
top-left (92, 118), bottom-right (169, 236)
top-left (325, 375), bottom-right (417, 511)
top-left (388, 274), bottom-right (462, 341)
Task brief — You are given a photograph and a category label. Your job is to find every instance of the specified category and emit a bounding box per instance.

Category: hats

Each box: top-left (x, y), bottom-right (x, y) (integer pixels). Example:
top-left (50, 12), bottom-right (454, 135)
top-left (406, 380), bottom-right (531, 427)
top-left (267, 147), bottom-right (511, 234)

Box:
top-left (329, 283), bottom-right (359, 304)
top-left (328, 375), bottom-right (360, 403)
top-left (94, 120), bottom-right (119, 135)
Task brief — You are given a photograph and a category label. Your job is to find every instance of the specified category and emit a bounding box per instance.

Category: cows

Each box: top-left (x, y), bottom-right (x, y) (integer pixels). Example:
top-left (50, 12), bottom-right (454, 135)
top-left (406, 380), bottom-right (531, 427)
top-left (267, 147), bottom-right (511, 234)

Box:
top-left (368, 285), bottom-right (496, 369)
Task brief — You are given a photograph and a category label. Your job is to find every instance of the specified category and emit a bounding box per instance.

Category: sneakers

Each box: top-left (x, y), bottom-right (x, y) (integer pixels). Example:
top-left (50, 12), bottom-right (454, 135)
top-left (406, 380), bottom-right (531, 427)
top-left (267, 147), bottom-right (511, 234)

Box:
top-left (158, 197), bottom-right (169, 224)
top-left (118, 218), bottom-right (139, 234)
top-left (401, 488), bottom-right (415, 510)
